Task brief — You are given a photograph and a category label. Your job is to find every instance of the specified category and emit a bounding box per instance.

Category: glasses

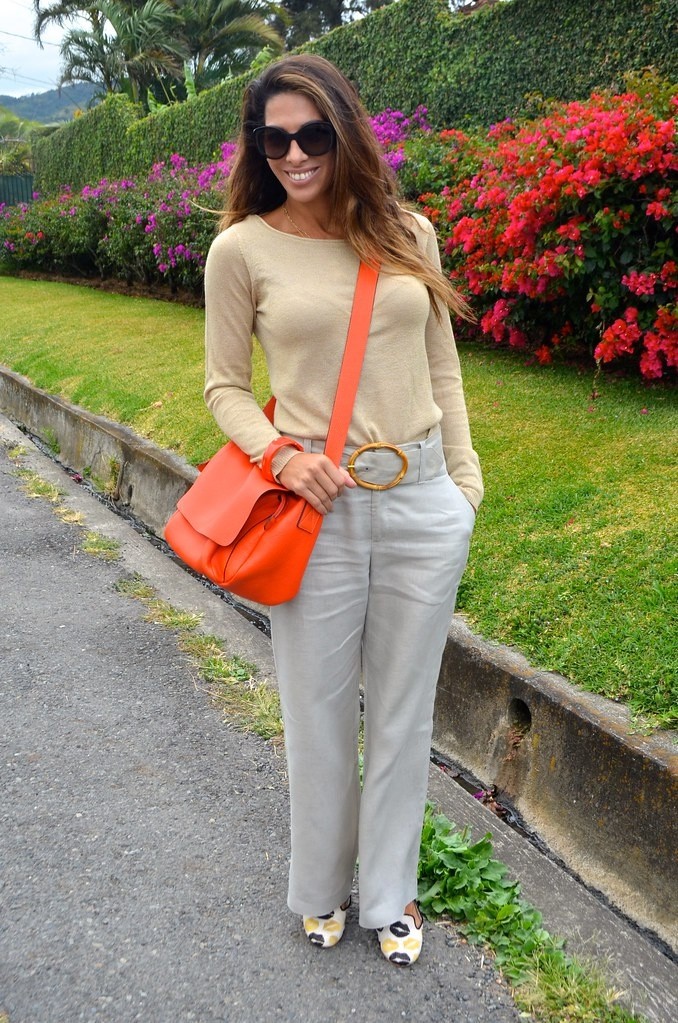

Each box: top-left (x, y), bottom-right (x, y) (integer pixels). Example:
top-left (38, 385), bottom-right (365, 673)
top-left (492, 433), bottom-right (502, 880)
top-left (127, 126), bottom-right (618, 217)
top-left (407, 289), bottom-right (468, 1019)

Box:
top-left (251, 120), bottom-right (337, 160)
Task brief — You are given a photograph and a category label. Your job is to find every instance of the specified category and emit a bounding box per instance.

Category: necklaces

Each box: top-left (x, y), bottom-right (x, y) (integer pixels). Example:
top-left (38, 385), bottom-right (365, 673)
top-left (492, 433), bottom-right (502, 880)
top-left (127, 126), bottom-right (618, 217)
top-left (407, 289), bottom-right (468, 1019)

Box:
top-left (283, 204), bottom-right (310, 240)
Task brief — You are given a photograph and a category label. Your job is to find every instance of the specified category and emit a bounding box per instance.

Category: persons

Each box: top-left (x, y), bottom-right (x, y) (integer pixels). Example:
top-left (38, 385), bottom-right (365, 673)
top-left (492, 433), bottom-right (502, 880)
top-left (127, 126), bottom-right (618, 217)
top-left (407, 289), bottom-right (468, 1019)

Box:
top-left (203, 52), bottom-right (483, 963)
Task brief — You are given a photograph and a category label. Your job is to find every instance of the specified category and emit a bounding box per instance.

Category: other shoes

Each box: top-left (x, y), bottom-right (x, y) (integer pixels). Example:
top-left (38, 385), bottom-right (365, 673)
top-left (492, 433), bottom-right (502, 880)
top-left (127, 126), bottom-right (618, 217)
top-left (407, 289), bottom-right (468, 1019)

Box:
top-left (302, 894), bottom-right (352, 948)
top-left (376, 901), bottom-right (425, 968)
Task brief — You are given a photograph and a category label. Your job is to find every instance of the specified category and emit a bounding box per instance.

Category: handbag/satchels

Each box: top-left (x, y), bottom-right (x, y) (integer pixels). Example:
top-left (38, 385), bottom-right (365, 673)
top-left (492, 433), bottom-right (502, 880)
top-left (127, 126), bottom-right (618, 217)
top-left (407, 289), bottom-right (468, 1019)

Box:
top-left (162, 438), bottom-right (325, 607)
